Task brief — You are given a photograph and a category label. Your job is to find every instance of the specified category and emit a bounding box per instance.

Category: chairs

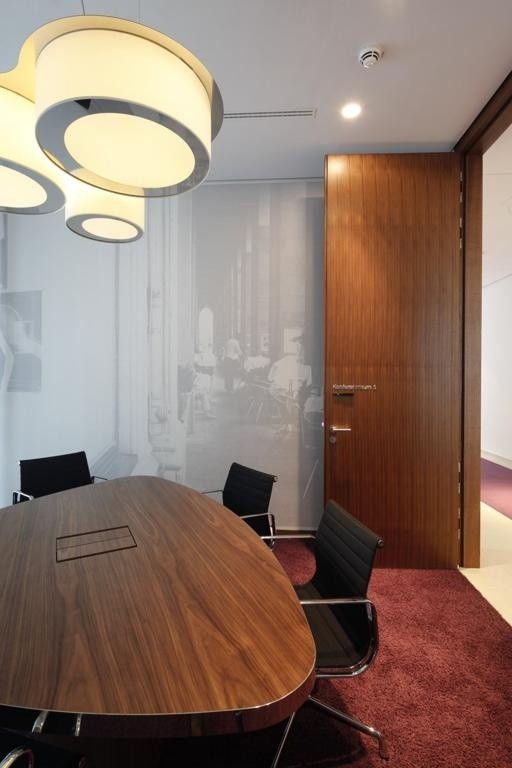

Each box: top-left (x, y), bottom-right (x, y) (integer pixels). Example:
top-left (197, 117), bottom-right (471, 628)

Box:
top-left (200, 462), bottom-right (278, 551)
top-left (215, 358), bottom-right (325, 497)
top-left (13, 451), bottom-right (108, 505)
top-left (0, 707), bottom-right (82, 767)
top-left (259, 499), bottom-right (391, 767)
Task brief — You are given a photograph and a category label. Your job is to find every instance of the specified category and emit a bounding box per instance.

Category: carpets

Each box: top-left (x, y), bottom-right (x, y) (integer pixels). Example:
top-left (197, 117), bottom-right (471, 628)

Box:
top-left (9, 532), bottom-right (512, 768)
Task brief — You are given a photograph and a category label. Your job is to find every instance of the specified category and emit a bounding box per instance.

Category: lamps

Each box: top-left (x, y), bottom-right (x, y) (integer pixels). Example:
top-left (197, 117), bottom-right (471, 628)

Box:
top-left (0, 2), bottom-right (224, 244)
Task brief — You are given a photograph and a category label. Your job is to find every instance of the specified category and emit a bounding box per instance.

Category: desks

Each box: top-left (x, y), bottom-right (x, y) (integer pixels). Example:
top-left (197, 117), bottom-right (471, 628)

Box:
top-left (1, 476), bottom-right (316, 768)
